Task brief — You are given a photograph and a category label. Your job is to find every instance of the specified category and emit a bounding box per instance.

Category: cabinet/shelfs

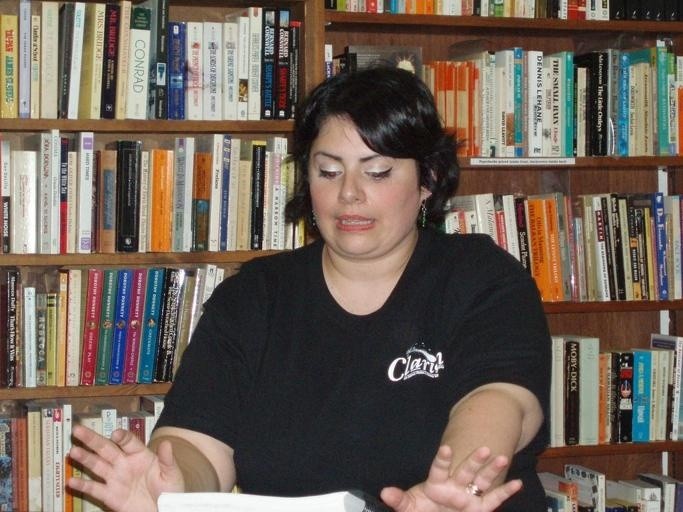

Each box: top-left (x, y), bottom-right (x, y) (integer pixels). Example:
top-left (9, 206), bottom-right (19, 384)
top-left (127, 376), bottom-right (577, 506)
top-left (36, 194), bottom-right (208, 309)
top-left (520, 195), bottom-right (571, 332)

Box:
top-left (0, 0), bottom-right (313, 512)
top-left (317, 0), bottom-right (683, 512)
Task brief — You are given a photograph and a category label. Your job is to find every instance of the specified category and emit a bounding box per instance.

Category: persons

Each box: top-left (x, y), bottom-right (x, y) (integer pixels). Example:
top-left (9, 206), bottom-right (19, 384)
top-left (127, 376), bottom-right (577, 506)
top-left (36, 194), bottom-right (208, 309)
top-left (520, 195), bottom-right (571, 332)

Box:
top-left (67, 65), bottom-right (553, 512)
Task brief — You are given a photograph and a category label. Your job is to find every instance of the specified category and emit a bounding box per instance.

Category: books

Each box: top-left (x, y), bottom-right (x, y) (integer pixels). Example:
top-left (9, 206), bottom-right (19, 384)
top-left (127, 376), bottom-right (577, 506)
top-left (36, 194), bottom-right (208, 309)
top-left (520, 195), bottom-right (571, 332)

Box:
top-left (501, 191), bottom-right (682, 302)
top-left (420, 38), bottom-right (682, 159)
top-left (538, 451), bottom-right (681, 512)
top-left (2, 265), bottom-right (225, 387)
top-left (324, 43), bottom-right (424, 78)
top-left (443, 190), bottom-right (506, 253)
top-left (551, 334), bottom-right (681, 448)
top-left (1, 397), bottom-right (164, 511)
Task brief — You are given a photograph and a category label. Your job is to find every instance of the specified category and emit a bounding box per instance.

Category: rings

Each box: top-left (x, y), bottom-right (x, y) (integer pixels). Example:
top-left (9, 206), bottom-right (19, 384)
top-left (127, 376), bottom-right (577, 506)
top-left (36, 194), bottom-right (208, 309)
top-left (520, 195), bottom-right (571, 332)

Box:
top-left (465, 483), bottom-right (485, 501)
